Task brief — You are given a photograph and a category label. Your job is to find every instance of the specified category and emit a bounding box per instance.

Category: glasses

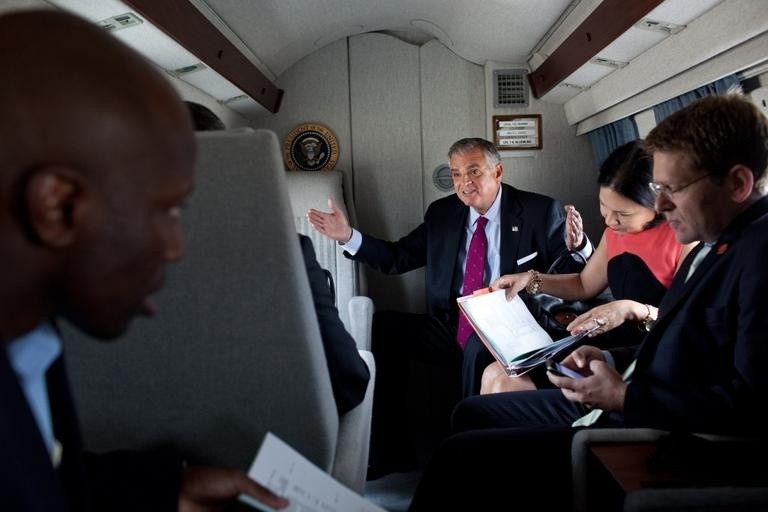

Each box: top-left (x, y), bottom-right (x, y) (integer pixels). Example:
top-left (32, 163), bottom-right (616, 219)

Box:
top-left (647, 172), bottom-right (706, 198)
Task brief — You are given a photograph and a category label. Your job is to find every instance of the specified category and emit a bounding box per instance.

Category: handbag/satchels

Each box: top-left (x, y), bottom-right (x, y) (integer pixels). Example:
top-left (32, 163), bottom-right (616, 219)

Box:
top-left (530, 250), bottom-right (612, 339)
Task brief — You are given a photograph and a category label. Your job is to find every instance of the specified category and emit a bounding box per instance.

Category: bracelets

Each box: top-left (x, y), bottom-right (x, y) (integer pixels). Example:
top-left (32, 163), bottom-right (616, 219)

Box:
top-left (526, 269), bottom-right (543, 296)
top-left (642, 303), bottom-right (653, 327)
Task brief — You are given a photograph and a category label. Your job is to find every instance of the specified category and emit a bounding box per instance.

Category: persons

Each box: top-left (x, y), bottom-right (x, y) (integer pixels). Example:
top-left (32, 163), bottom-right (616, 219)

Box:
top-left (1, 6), bottom-right (293, 511)
top-left (404, 80), bottom-right (768, 510)
top-left (306, 135), bottom-right (598, 482)
top-left (173, 99), bottom-right (372, 419)
top-left (476, 138), bottom-right (702, 401)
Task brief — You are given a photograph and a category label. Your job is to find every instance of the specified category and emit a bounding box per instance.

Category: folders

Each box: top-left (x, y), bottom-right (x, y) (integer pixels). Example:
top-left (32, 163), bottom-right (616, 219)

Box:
top-left (455, 287), bottom-right (602, 377)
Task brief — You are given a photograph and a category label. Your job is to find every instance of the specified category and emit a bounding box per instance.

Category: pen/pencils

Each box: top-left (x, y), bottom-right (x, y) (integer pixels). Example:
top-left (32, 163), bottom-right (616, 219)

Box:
top-left (510, 347), bottom-right (545, 363)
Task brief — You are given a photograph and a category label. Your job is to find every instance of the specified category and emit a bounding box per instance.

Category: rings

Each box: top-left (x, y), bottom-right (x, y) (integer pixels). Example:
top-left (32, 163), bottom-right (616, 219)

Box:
top-left (591, 318), bottom-right (598, 325)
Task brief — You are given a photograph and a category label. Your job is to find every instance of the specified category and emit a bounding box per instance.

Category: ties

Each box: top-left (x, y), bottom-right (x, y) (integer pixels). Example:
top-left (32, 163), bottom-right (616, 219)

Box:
top-left (455, 217), bottom-right (487, 352)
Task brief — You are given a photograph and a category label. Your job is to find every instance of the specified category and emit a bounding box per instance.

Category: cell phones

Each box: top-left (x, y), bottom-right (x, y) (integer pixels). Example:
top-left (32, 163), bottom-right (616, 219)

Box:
top-left (545, 359), bottom-right (586, 380)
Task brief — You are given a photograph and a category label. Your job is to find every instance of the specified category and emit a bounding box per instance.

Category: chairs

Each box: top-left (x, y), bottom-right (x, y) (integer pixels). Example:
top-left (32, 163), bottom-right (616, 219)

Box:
top-left (281, 163), bottom-right (375, 352)
top-left (56, 123), bottom-right (376, 499)
